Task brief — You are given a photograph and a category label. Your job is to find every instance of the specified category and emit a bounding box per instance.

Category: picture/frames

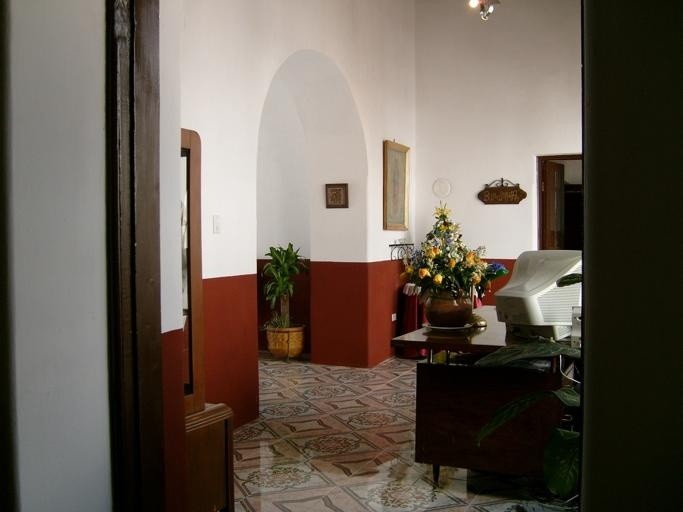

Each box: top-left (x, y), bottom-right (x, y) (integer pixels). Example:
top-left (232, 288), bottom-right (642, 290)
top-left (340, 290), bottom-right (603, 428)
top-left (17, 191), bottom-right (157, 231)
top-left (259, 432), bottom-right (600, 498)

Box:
top-left (383, 139), bottom-right (409, 231)
top-left (324, 183), bottom-right (350, 209)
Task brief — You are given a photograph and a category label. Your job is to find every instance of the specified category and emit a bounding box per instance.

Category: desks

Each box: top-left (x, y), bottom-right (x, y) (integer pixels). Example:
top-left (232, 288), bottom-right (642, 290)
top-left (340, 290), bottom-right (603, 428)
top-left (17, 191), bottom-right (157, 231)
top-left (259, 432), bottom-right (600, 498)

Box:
top-left (393, 304), bottom-right (584, 484)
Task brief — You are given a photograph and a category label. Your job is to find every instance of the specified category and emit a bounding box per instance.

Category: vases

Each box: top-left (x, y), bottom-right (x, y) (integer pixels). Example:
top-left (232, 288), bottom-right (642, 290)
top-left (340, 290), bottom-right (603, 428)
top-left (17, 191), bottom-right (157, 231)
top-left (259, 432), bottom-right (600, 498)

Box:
top-left (423, 290), bottom-right (475, 328)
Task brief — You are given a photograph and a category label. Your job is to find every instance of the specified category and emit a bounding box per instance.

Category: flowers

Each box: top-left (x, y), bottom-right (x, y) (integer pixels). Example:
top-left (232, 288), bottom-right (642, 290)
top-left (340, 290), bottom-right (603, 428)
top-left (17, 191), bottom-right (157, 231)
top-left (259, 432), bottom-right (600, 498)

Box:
top-left (392, 200), bottom-right (507, 295)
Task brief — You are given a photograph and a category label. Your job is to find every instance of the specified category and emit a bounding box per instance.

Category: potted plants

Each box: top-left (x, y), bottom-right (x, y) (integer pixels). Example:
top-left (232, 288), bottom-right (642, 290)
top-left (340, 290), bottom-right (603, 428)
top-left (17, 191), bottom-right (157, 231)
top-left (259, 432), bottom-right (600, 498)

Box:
top-left (256, 240), bottom-right (311, 361)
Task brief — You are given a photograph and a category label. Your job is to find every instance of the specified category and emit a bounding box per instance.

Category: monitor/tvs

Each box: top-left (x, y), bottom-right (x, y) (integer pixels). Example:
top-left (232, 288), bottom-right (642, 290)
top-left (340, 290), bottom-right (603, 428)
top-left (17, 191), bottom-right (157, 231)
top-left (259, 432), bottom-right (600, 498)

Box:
top-left (494, 249), bottom-right (583, 342)
